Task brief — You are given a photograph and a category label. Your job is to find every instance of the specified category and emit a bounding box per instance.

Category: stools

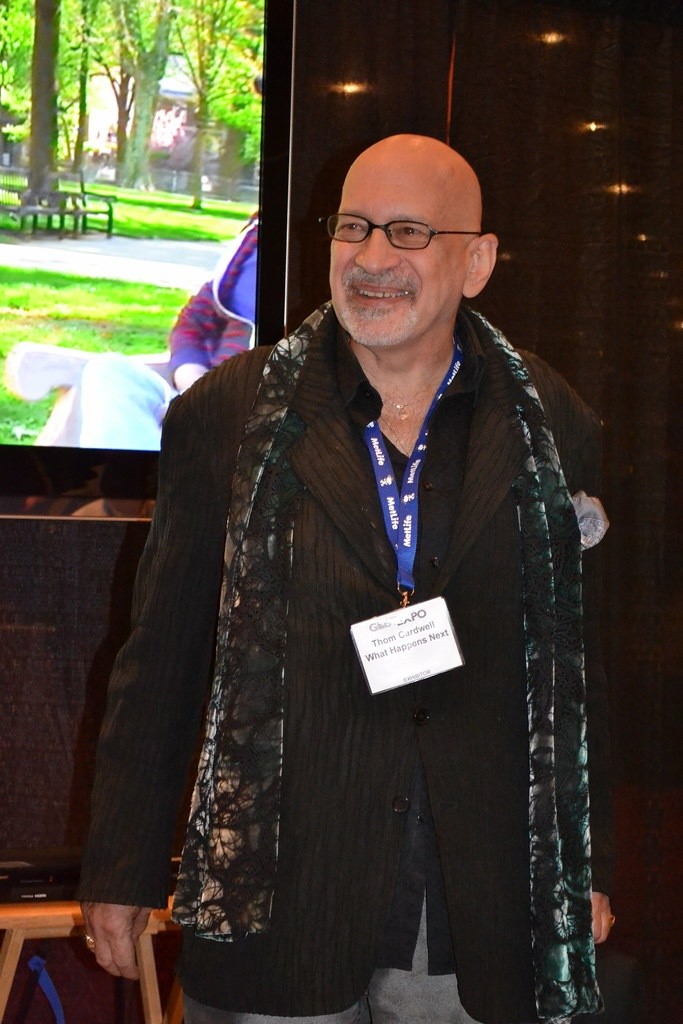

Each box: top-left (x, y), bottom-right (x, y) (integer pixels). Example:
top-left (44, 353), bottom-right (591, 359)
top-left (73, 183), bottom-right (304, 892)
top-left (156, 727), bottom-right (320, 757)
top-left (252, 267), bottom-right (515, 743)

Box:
top-left (0, 897), bottom-right (184, 1024)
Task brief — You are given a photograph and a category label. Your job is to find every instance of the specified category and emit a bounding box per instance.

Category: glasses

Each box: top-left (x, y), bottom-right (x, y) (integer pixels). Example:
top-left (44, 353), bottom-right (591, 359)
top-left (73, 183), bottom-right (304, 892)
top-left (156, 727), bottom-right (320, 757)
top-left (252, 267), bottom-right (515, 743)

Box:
top-left (318, 212), bottom-right (482, 249)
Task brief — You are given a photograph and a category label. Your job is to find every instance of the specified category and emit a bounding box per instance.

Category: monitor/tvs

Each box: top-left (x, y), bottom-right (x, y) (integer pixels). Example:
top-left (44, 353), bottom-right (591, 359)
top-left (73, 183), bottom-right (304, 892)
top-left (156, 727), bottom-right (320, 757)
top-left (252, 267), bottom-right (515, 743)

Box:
top-left (0, 0), bottom-right (300, 513)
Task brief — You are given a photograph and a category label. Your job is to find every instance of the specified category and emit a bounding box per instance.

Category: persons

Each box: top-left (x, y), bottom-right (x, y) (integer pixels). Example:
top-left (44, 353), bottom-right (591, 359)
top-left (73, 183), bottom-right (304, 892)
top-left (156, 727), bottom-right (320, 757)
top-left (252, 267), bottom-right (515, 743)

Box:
top-left (165, 209), bottom-right (258, 395)
top-left (78, 130), bottom-right (617, 1024)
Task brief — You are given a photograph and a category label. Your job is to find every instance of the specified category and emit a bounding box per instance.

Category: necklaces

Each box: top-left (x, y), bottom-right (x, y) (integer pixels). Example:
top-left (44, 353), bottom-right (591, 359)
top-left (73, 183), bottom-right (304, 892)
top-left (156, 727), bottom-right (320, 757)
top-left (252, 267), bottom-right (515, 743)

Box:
top-left (373, 388), bottom-right (427, 458)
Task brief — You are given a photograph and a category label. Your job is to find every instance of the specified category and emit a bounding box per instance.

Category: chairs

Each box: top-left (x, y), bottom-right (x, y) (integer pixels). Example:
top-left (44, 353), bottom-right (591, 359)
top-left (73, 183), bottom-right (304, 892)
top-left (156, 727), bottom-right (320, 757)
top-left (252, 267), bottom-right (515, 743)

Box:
top-left (13, 169), bottom-right (118, 238)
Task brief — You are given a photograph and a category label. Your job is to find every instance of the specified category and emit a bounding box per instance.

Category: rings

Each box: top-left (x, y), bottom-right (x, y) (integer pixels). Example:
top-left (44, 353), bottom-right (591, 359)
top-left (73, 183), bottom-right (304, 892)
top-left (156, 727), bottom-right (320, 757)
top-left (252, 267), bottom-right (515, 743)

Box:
top-left (610, 915), bottom-right (616, 926)
top-left (83, 932), bottom-right (95, 949)
